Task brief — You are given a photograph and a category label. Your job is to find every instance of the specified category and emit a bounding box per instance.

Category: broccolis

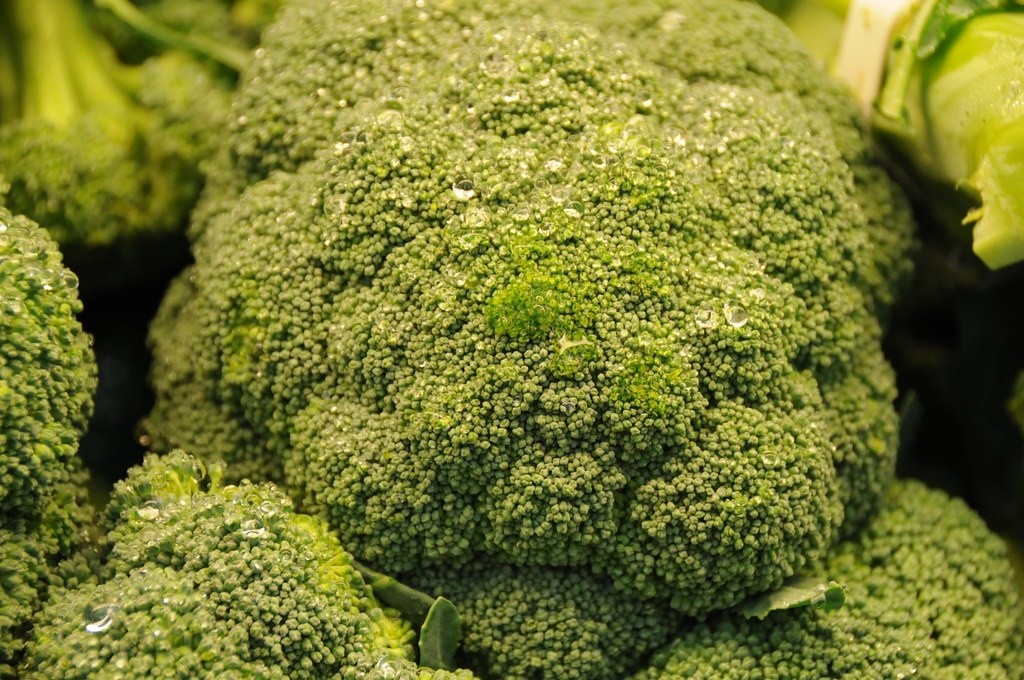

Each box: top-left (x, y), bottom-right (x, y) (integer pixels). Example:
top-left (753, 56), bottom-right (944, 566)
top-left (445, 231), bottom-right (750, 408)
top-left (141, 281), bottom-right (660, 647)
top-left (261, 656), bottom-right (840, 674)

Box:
top-left (0, 0), bottom-right (1024, 680)
